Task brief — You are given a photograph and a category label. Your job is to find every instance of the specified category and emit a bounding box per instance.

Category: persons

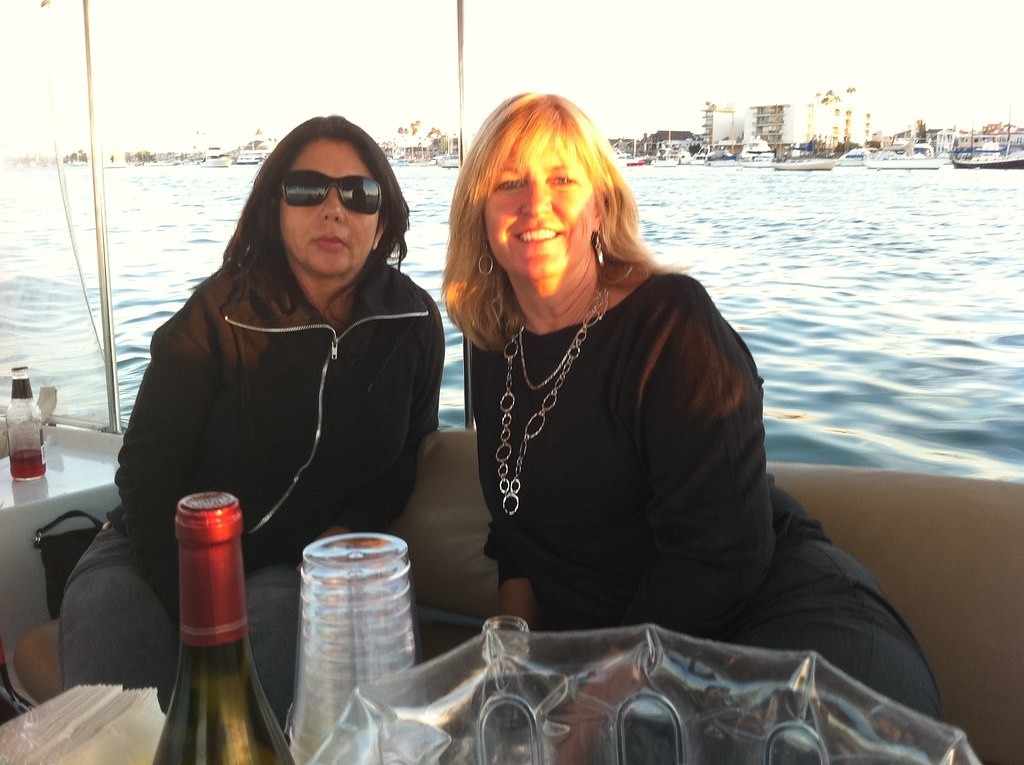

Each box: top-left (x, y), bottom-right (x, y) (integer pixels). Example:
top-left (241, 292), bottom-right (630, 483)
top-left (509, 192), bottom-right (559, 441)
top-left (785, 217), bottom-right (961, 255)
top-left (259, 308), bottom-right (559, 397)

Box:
top-left (59, 115), bottom-right (445, 729)
top-left (443, 92), bottom-right (942, 724)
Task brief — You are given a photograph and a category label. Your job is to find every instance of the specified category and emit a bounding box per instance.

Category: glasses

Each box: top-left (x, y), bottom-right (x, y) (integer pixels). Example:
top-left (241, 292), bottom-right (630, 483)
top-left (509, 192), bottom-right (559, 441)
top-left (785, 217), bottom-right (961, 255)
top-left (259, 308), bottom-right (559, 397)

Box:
top-left (278, 170), bottom-right (382, 214)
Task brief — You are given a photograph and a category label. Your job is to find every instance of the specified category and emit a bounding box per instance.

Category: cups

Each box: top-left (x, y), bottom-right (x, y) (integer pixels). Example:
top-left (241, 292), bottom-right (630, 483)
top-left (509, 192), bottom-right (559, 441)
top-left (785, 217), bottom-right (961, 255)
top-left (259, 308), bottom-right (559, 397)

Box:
top-left (285, 533), bottom-right (416, 765)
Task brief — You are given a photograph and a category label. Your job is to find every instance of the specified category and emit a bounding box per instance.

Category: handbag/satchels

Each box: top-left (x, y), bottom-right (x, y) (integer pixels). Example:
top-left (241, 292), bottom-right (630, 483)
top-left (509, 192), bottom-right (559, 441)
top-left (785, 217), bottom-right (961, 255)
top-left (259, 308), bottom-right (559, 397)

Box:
top-left (33, 511), bottom-right (105, 620)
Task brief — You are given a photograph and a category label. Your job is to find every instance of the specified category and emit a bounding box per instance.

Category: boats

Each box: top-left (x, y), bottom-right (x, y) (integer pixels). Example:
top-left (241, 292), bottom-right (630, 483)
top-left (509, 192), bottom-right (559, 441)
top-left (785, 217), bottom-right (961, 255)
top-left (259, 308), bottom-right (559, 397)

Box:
top-left (388, 133), bottom-right (1024, 171)
top-left (234, 147), bottom-right (275, 166)
top-left (200, 141), bottom-right (233, 169)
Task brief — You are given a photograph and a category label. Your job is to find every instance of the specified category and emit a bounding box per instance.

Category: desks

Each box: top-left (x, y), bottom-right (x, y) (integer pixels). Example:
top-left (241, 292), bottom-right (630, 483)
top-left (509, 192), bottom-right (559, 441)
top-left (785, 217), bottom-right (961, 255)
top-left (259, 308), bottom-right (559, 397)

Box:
top-left (0, 445), bottom-right (122, 666)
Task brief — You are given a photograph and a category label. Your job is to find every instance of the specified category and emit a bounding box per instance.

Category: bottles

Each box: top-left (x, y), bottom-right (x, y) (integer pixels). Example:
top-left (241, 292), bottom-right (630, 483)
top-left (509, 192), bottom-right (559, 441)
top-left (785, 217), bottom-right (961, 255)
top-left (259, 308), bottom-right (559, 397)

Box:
top-left (6, 366), bottom-right (46, 482)
top-left (151, 492), bottom-right (297, 765)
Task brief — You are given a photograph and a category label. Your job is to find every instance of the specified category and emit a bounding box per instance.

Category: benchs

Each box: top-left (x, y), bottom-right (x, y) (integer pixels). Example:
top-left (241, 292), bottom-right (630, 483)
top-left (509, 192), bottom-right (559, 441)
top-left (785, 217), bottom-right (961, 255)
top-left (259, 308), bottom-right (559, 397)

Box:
top-left (15, 428), bottom-right (1024, 765)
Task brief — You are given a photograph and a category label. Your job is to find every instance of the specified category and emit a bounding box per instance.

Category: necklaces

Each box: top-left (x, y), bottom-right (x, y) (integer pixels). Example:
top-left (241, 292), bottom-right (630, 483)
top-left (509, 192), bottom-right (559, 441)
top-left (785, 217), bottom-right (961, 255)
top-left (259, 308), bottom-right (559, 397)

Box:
top-left (496, 286), bottom-right (608, 515)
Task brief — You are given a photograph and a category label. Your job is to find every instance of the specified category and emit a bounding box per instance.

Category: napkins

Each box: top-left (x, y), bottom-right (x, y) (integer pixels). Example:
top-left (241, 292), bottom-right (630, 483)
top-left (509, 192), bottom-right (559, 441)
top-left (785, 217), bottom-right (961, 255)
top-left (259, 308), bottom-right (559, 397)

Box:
top-left (0, 683), bottom-right (167, 765)
